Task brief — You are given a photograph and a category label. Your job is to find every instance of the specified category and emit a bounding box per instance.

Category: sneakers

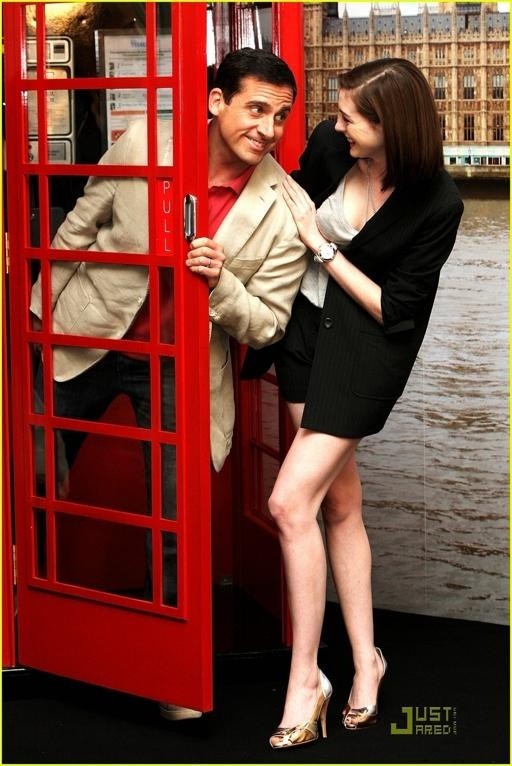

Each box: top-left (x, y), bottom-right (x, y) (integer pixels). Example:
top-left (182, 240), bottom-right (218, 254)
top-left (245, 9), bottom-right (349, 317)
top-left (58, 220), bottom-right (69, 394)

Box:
top-left (158, 700), bottom-right (202, 722)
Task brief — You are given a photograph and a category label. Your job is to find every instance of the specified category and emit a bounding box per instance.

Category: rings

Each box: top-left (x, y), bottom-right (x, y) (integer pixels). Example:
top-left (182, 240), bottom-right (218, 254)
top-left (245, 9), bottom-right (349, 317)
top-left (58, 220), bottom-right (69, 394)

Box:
top-left (208, 258), bottom-right (211, 267)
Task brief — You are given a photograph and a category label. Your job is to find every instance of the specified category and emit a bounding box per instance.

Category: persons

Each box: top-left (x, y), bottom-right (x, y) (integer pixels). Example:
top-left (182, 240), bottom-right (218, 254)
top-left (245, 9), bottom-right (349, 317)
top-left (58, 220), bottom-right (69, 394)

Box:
top-left (262, 56), bottom-right (465, 750)
top-left (28, 47), bottom-right (315, 610)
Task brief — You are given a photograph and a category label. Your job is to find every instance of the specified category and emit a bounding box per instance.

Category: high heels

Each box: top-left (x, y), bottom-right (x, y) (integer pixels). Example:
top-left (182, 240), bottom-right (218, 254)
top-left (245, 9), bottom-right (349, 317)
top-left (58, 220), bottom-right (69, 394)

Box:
top-left (341, 648), bottom-right (387, 733)
top-left (270, 670), bottom-right (333, 750)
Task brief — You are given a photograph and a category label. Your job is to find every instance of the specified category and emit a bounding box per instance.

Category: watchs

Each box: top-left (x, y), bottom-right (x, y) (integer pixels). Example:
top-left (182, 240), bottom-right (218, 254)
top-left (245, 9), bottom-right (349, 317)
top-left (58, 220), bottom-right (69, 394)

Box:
top-left (313, 242), bottom-right (340, 263)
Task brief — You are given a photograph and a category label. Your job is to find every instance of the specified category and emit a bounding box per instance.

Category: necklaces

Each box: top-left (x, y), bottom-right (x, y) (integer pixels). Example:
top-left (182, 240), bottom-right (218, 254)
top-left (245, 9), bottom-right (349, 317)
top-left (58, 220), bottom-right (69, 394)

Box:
top-left (365, 158), bottom-right (376, 223)
